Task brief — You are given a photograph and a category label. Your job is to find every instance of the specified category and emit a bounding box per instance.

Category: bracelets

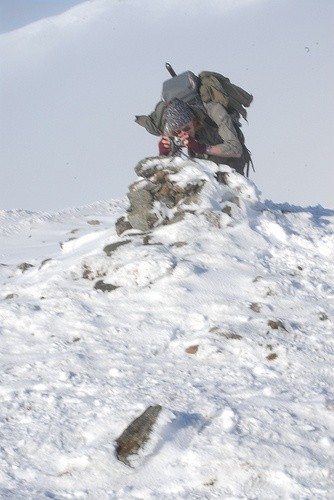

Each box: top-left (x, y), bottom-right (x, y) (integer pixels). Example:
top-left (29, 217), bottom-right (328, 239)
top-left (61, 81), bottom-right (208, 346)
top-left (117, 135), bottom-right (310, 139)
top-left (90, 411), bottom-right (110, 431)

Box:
top-left (205, 143), bottom-right (210, 154)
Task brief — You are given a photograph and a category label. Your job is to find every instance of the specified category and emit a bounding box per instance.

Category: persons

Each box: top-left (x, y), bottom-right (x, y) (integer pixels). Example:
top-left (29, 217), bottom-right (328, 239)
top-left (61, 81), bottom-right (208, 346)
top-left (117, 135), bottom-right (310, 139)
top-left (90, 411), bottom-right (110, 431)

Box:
top-left (159, 98), bottom-right (245, 177)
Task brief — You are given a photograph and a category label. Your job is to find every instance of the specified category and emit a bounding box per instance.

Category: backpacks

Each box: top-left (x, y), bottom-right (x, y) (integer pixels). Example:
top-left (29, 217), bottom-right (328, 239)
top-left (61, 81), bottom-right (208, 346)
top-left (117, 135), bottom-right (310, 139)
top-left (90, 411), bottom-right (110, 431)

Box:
top-left (161, 70), bottom-right (200, 105)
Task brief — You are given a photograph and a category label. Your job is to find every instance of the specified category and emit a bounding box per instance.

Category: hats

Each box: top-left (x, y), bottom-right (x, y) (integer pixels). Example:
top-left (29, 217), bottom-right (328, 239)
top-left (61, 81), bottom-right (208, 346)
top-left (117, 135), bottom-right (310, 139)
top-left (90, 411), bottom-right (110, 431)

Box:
top-left (162, 98), bottom-right (194, 131)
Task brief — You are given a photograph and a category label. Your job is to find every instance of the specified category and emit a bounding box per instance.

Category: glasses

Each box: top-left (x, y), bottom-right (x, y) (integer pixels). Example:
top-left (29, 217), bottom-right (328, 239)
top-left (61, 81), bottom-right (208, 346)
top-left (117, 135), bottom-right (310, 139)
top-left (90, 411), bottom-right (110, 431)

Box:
top-left (172, 124), bottom-right (193, 134)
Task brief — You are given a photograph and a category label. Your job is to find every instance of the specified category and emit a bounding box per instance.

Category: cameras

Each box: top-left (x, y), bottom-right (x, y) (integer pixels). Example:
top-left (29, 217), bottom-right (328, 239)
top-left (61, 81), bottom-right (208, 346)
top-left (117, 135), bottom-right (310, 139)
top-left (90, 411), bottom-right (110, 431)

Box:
top-left (169, 136), bottom-right (185, 147)
